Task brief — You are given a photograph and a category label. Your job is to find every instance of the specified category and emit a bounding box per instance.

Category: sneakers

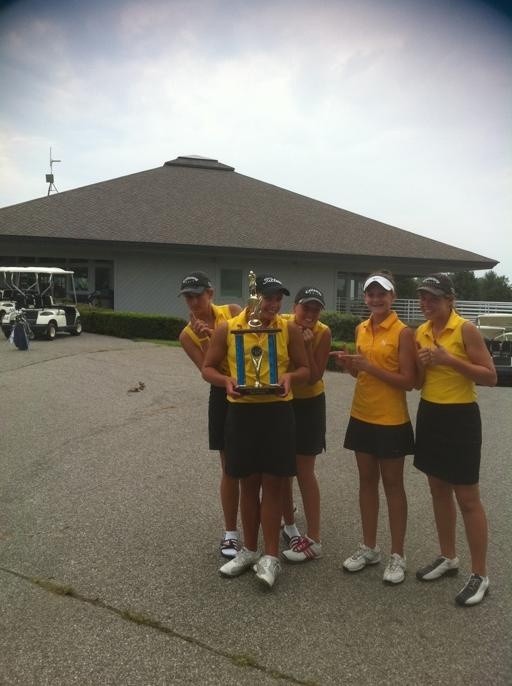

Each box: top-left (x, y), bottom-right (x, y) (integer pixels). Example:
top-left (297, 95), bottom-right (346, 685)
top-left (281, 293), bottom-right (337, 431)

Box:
top-left (416, 555), bottom-right (459, 581)
top-left (252, 554), bottom-right (282, 587)
top-left (219, 546), bottom-right (263, 578)
top-left (455, 573), bottom-right (490, 607)
top-left (342, 544), bottom-right (382, 573)
top-left (382, 552), bottom-right (408, 585)
top-left (281, 534), bottom-right (324, 563)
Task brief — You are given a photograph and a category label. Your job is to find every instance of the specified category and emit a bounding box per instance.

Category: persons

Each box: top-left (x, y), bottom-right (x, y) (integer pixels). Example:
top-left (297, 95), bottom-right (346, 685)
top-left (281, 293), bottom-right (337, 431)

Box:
top-left (413, 273), bottom-right (497, 607)
top-left (279, 285), bottom-right (332, 564)
top-left (176, 273), bottom-right (301, 559)
top-left (200, 276), bottom-right (311, 589)
top-left (329, 269), bottom-right (415, 586)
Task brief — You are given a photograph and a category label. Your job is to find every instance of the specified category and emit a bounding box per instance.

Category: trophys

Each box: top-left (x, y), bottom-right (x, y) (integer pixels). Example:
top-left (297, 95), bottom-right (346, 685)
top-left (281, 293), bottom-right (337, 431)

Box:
top-left (230, 270), bottom-right (285, 396)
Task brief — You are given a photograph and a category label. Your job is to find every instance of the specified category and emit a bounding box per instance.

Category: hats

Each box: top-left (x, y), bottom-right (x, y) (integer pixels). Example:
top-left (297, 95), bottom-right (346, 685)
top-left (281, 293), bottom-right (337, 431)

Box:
top-left (256, 274), bottom-right (292, 297)
top-left (363, 275), bottom-right (394, 291)
top-left (294, 286), bottom-right (326, 307)
top-left (416, 273), bottom-right (454, 296)
top-left (176, 271), bottom-right (212, 297)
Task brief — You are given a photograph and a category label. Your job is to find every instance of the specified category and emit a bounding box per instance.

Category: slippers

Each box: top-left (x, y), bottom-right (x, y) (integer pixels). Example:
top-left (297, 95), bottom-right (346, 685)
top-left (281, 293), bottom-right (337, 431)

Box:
top-left (282, 532), bottom-right (302, 549)
top-left (221, 537), bottom-right (239, 558)
